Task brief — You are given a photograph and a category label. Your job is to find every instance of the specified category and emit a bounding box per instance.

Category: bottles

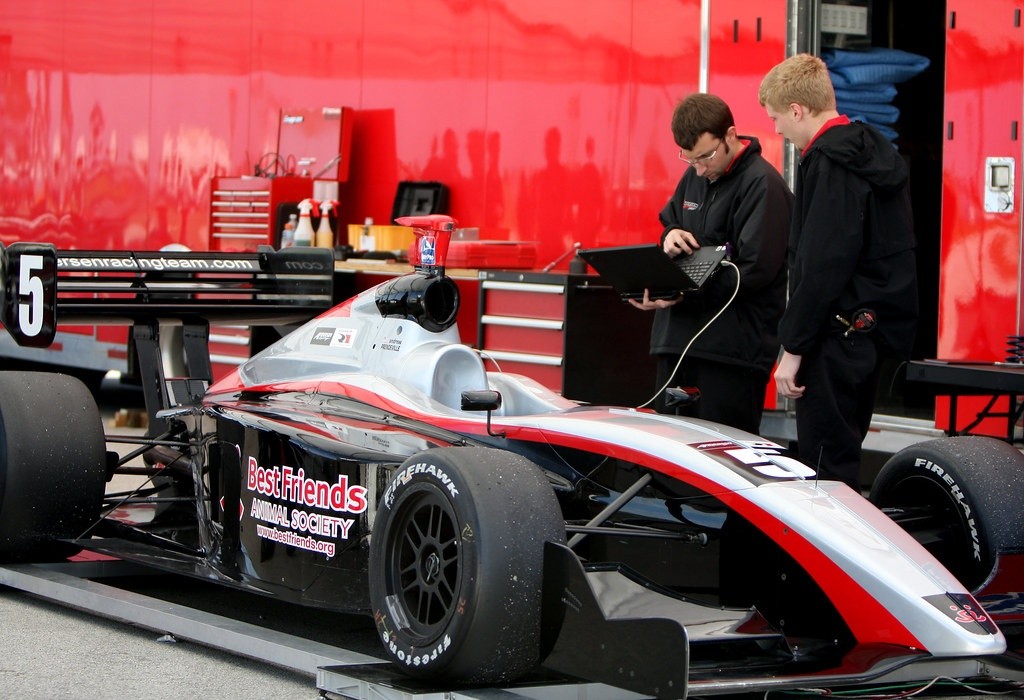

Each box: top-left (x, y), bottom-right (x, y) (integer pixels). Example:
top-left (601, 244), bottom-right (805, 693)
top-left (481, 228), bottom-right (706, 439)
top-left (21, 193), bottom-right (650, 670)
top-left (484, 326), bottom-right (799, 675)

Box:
top-left (361, 218), bottom-right (375, 251)
top-left (280, 223), bottom-right (293, 250)
top-left (288, 214), bottom-right (298, 229)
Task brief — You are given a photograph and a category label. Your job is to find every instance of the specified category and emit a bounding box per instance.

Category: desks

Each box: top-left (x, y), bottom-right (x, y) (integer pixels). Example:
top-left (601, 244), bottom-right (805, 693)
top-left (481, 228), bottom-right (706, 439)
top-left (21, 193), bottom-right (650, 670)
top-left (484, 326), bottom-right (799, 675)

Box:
top-left (906, 359), bottom-right (1024, 447)
top-left (334, 259), bottom-right (480, 281)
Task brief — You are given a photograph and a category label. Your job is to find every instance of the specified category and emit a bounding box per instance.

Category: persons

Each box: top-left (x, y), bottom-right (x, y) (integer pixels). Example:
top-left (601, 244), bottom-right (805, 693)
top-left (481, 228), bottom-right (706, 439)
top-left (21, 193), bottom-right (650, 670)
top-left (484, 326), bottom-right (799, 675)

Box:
top-left (757, 53), bottom-right (922, 491)
top-left (12, 154), bottom-right (89, 218)
top-left (146, 157), bottom-right (203, 253)
top-left (628, 91), bottom-right (796, 438)
top-left (423, 127), bottom-right (671, 273)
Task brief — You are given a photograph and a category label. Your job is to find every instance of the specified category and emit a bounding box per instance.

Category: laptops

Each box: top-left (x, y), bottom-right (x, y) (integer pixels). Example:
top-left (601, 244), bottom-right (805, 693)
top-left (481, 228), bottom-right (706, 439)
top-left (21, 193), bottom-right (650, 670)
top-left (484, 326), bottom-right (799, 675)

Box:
top-left (578, 242), bottom-right (728, 303)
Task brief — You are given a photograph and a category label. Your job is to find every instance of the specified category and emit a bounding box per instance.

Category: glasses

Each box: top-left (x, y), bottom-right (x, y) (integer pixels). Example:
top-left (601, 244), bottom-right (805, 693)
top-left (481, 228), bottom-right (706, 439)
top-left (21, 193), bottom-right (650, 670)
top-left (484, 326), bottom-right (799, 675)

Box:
top-left (678, 137), bottom-right (724, 167)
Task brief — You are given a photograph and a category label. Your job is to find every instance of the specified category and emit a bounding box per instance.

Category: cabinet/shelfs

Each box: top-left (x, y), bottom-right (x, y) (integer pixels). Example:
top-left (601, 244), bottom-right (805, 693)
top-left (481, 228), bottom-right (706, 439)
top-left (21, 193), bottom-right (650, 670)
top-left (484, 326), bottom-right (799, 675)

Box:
top-left (476, 270), bottom-right (650, 405)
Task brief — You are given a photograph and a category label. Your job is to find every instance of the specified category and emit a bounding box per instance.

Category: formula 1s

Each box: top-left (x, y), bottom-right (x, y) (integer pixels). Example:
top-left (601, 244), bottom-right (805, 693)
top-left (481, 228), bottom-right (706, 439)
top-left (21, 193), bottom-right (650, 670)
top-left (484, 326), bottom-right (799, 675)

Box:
top-left (0, 212), bottom-right (1024, 700)
